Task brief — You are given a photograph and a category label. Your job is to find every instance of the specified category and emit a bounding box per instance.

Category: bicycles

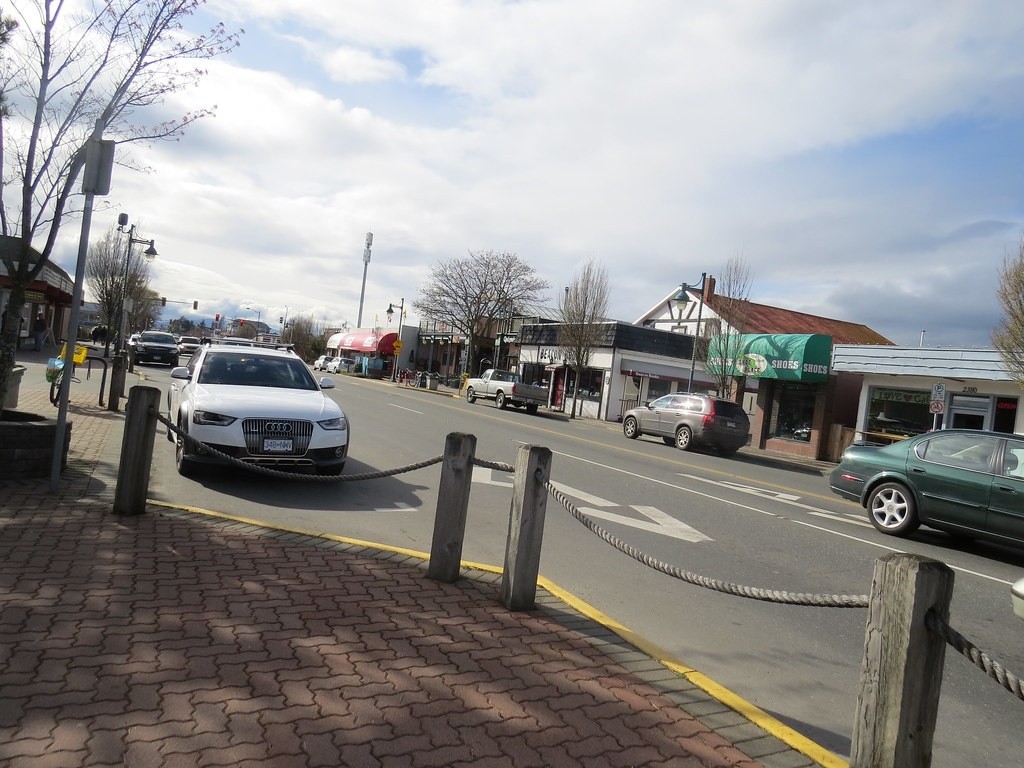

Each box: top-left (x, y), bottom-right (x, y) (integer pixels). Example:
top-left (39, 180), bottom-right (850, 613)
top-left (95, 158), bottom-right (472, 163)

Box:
top-left (46, 337), bottom-right (98, 403)
top-left (401, 368), bottom-right (452, 388)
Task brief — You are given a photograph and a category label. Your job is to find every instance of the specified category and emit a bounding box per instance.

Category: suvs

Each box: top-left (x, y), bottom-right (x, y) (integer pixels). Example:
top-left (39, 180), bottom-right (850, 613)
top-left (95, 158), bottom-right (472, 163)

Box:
top-left (622, 391), bottom-right (750, 457)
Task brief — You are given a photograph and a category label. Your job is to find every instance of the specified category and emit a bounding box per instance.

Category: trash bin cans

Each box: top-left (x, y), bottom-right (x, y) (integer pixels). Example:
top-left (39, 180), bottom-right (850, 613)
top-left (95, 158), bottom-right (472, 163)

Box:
top-left (417, 373), bottom-right (428, 388)
top-left (426, 375), bottom-right (439, 390)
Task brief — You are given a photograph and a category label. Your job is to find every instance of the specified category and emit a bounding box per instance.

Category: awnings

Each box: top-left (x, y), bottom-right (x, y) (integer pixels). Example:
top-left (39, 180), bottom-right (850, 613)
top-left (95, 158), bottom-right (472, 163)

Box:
top-left (706, 334), bottom-right (834, 383)
top-left (327, 332), bottom-right (349, 350)
top-left (339, 332), bottom-right (398, 355)
top-left (545, 363), bottom-right (567, 372)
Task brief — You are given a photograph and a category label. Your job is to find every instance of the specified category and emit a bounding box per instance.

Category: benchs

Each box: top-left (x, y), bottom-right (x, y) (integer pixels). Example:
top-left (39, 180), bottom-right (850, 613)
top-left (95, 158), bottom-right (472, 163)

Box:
top-left (203, 364), bottom-right (256, 380)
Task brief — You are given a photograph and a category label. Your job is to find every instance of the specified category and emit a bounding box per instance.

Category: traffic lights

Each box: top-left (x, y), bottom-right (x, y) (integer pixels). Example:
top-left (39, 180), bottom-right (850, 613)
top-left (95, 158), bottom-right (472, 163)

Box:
top-left (280, 317), bottom-right (283, 323)
top-left (241, 319), bottom-right (243, 326)
top-left (216, 314), bottom-right (219, 320)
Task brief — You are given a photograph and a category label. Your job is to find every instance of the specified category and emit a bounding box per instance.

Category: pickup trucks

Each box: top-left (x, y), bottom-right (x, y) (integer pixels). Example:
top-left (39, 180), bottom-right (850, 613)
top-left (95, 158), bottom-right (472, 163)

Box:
top-left (466, 368), bottom-right (549, 415)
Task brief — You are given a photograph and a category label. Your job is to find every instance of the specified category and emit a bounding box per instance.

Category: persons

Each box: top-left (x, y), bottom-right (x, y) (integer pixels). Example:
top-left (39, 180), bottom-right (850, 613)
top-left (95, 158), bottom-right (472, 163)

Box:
top-left (30, 314), bottom-right (47, 352)
top-left (99, 326), bottom-right (107, 345)
top-left (92, 328), bottom-right (99, 344)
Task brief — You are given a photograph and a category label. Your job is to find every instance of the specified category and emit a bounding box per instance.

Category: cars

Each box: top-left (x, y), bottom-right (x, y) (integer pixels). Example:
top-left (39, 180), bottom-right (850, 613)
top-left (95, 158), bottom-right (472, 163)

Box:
top-left (135, 329), bottom-right (181, 369)
top-left (313, 354), bottom-right (335, 372)
top-left (200, 335), bottom-right (221, 346)
top-left (326, 357), bottom-right (355, 374)
top-left (167, 342), bottom-right (351, 477)
top-left (177, 335), bottom-right (201, 356)
top-left (127, 333), bottom-right (142, 348)
top-left (829, 428), bottom-right (1024, 561)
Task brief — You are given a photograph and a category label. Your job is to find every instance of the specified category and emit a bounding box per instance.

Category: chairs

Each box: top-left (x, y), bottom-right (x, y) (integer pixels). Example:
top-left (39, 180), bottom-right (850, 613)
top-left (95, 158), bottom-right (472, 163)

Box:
top-left (209, 357), bottom-right (229, 381)
top-left (1003, 454), bottom-right (1018, 476)
top-left (256, 363), bottom-right (277, 381)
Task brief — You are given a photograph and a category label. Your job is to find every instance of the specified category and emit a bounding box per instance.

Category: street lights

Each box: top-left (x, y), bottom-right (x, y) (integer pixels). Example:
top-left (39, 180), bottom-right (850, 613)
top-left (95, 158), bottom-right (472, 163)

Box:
top-left (107, 213), bottom-right (160, 411)
top-left (358, 231), bottom-right (374, 328)
top-left (386, 297), bottom-right (405, 382)
top-left (246, 307), bottom-right (260, 341)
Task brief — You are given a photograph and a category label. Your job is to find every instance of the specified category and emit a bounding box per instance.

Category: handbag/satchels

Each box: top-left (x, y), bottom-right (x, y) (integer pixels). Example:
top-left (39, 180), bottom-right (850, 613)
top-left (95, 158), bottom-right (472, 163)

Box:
top-left (45, 355), bottom-right (64, 383)
